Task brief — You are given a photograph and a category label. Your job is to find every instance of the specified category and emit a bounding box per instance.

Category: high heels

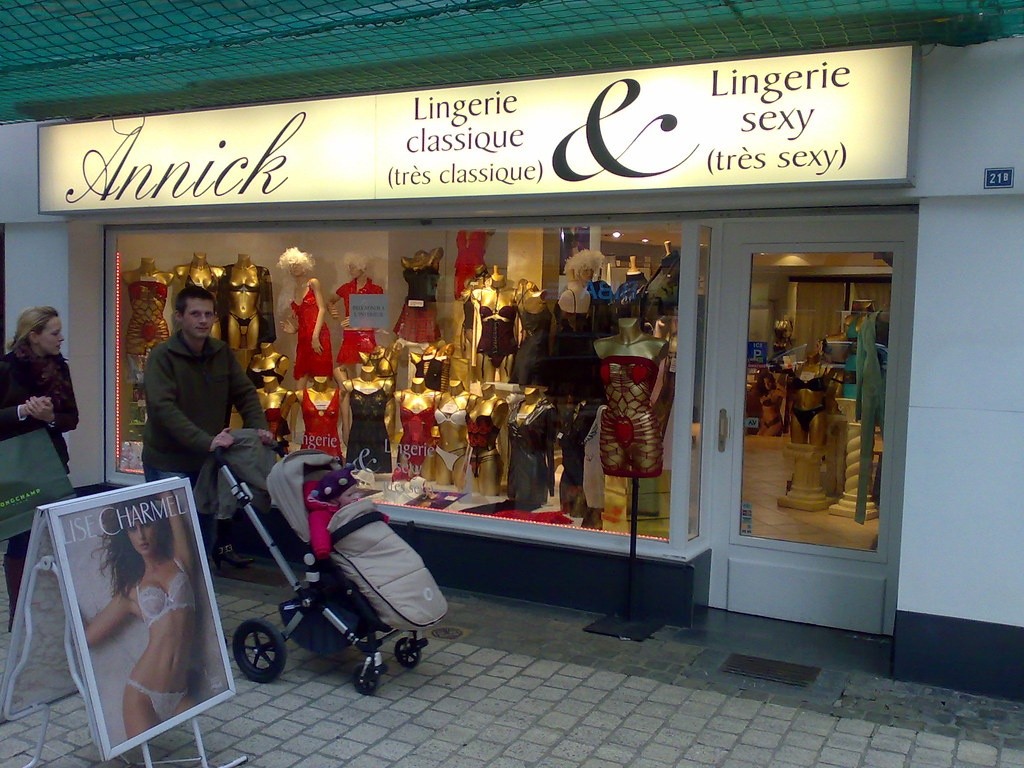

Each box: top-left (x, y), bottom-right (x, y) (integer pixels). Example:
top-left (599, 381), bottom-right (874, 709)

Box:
top-left (212, 546), bottom-right (254, 569)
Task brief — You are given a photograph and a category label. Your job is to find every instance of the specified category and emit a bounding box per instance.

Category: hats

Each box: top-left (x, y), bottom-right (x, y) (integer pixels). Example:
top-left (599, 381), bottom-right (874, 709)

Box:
top-left (313, 464), bottom-right (357, 504)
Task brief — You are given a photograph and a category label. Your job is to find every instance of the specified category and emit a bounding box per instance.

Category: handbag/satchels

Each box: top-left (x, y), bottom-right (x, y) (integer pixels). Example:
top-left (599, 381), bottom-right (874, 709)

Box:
top-left (0, 429), bottom-right (79, 543)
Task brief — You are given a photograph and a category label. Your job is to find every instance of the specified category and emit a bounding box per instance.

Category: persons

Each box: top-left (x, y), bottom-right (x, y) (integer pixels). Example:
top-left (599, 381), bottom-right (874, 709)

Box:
top-left (142, 285), bottom-right (272, 644)
top-left (83, 491), bottom-right (197, 739)
top-left (303, 469), bottom-right (389, 559)
top-left (0, 306), bottom-right (79, 632)
top-left (120, 230), bottom-right (833, 529)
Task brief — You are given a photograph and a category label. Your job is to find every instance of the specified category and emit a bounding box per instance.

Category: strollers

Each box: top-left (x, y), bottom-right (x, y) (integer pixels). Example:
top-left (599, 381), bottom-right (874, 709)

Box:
top-left (212, 428), bottom-right (450, 698)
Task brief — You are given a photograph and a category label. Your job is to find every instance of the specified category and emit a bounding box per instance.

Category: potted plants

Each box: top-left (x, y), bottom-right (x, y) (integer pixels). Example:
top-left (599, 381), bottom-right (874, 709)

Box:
top-left (827, 335), bottom-right (853, 363)
